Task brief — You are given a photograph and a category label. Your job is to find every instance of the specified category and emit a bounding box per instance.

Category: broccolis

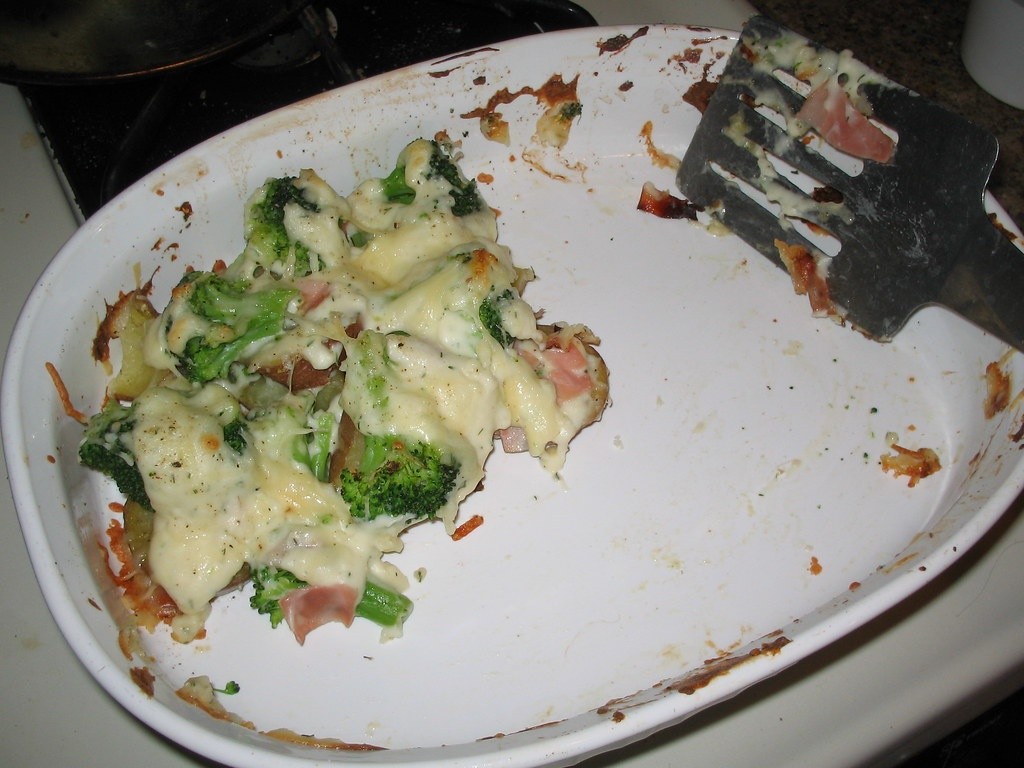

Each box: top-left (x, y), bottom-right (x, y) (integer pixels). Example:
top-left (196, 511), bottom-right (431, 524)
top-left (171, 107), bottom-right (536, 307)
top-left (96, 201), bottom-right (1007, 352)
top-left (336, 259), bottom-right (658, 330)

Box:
top-left (75, 141), bottom-right (516, 629)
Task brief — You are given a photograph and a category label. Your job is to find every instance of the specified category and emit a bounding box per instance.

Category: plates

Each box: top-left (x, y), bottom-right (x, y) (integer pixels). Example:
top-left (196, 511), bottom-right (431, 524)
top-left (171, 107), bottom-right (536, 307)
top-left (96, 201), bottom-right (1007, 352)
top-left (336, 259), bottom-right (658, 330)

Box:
top-left (1, 22), bottom-right (1024, 768)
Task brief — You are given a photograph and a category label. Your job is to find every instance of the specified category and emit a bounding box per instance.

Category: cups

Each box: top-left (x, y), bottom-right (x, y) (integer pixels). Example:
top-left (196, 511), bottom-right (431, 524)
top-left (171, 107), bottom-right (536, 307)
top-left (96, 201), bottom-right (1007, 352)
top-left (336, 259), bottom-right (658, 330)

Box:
top-left (961, 0), bottom-right (1024, 111)
